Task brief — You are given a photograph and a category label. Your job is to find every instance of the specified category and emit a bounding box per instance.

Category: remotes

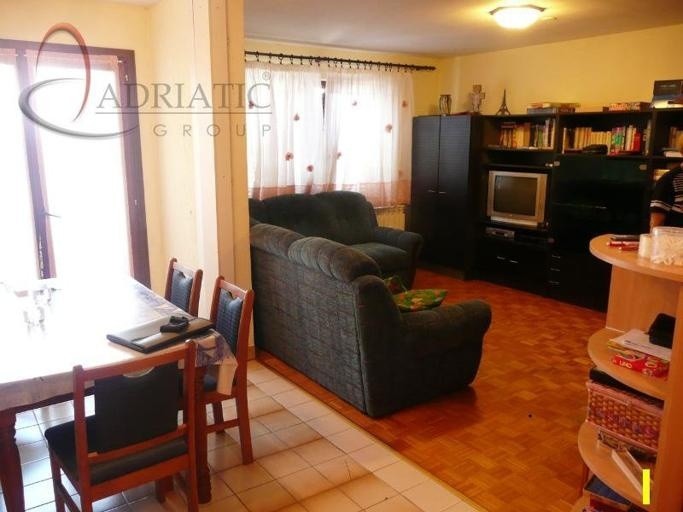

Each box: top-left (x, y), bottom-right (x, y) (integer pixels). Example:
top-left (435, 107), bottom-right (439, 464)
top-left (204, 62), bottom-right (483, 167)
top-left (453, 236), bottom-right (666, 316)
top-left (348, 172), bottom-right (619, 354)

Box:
top-left (613, 234), bottom-right (641, 241)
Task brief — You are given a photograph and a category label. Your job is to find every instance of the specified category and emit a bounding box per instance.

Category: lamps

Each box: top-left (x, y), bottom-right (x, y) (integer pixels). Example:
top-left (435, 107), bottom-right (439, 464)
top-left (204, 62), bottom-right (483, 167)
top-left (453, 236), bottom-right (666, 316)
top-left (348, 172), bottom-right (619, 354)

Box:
top-left (489, 4), bottom-right (545, 30)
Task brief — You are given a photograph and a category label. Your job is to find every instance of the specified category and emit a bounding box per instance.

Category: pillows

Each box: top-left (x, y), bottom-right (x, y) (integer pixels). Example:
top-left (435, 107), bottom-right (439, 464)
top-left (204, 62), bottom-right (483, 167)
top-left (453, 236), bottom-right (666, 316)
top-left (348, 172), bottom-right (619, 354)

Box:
top-left (382, 274), bottom-right (448, 313)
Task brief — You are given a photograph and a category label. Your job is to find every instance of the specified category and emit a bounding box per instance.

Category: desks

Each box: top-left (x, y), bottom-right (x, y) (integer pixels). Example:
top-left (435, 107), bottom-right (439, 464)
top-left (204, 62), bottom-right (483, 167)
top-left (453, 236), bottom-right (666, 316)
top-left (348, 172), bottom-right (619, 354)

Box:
top-left (1, 277), bottom-right (238, 511)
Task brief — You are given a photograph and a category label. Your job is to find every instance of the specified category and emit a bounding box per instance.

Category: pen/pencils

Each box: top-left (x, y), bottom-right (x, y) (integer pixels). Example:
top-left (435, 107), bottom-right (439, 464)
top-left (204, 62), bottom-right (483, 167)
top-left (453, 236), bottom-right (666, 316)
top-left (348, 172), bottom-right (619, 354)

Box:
top-left (605, 241), bottom-right (639, 250)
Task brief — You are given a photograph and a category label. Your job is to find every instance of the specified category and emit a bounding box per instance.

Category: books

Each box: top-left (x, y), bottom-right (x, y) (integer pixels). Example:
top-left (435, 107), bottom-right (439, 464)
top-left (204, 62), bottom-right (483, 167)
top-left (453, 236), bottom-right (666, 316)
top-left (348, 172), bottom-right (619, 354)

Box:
top-left (497, 118), bottom-right (651, 156)
top-left (608, 102), bottom-right (651, 112)
top-left (605, 329), bottom-right (671, 364)
top-left (529, 101), bottom-right (580, 108)
top-left (526, 107), bottom-right (575, 114)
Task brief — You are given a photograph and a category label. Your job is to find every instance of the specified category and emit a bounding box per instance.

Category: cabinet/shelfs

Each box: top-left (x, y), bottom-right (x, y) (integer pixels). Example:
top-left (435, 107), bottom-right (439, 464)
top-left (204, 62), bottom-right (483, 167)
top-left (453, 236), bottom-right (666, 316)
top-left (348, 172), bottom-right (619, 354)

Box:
top-left (410, 104), bottom-right (683, 313)
top-left (578, 234), bottom-right (683, 512)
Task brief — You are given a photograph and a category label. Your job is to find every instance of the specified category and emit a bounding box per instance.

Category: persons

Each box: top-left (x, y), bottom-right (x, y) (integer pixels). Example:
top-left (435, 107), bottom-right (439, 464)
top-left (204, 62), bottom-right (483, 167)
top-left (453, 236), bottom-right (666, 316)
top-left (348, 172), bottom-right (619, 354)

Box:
top-left (649, 166), bottom-right (682, 235)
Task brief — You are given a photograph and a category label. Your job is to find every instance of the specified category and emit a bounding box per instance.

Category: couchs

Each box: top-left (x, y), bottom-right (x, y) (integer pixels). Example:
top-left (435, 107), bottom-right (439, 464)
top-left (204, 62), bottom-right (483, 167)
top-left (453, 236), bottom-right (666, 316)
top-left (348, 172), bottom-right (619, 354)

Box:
top-left (249, 191), bottom-right (491, 418)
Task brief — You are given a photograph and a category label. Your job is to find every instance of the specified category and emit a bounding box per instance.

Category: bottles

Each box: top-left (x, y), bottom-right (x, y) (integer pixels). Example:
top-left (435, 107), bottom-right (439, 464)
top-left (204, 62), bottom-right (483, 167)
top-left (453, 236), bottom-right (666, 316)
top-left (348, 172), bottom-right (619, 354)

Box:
top-left (438, 93), bottom-right (453, 115)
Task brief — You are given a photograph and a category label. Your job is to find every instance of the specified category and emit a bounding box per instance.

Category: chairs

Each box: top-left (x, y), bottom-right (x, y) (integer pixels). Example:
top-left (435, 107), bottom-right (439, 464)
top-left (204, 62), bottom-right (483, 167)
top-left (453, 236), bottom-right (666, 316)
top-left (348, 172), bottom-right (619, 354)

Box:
top-left (44, 258), bottom-right (254, 512)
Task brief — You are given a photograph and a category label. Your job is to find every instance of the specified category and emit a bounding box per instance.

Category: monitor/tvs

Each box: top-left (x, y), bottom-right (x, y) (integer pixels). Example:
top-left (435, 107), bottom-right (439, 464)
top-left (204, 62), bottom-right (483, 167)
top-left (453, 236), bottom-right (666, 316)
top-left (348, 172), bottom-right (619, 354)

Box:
top-left (486, 170), bottom-right (550, 226)
